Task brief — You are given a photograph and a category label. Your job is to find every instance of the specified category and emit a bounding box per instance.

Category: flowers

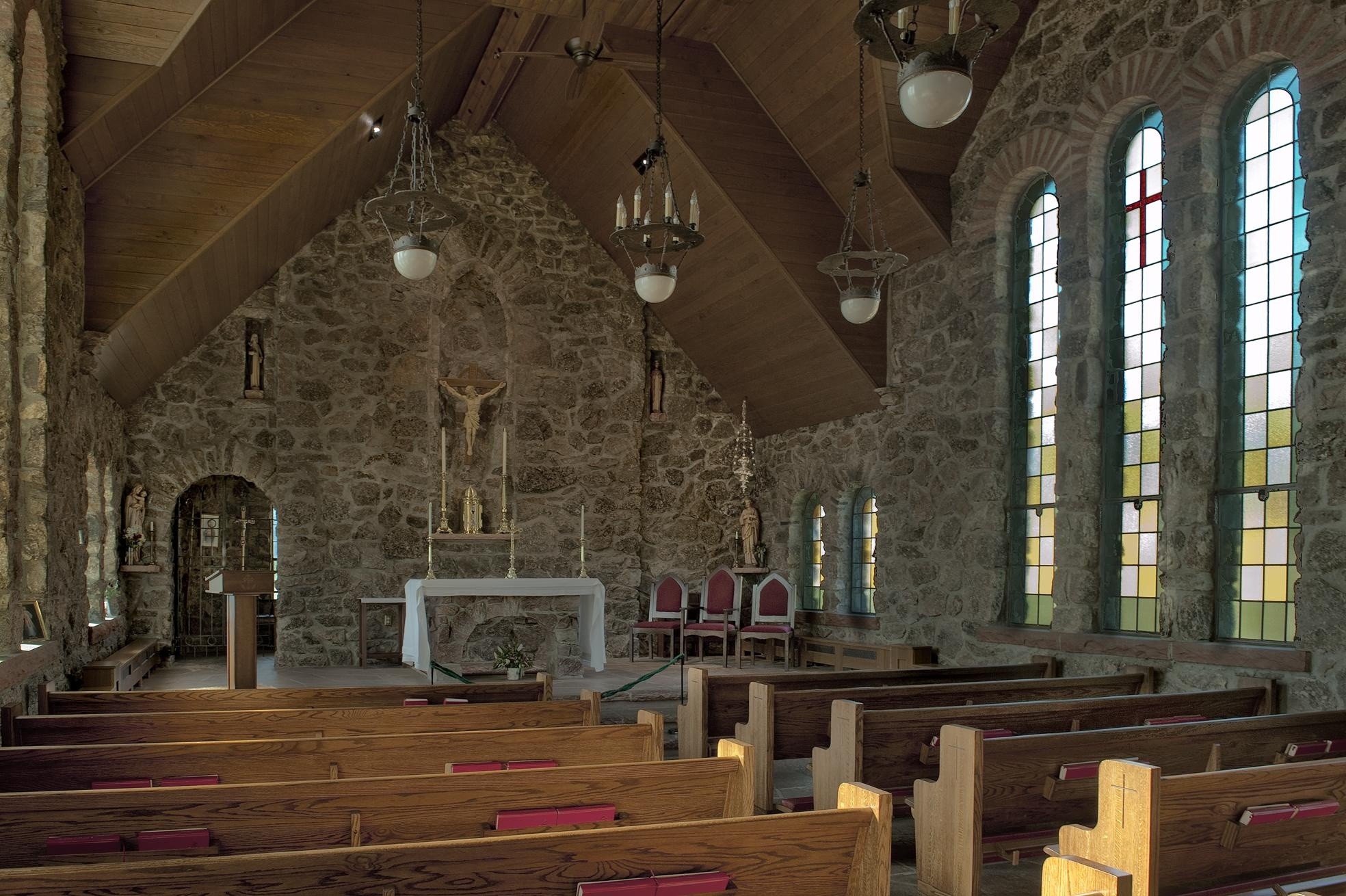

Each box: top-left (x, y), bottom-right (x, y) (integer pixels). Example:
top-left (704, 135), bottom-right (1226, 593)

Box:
top-left (121, 525), bottom-right (147, 550)
top-left (492, 641), bottom-right (534, 680)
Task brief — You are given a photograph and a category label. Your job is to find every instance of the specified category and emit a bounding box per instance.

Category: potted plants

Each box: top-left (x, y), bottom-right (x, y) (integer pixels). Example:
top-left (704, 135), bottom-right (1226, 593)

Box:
top-left (102, 579), bottom-right (121, 617)
top-left (752, 542), bottom-right (767, 568)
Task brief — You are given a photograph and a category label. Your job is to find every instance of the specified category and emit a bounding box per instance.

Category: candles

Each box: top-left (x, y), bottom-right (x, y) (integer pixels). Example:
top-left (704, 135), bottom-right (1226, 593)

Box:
top-left (581, 506), bottom-right (585, 538)
top-left (427, 427), bottom-right (446, 536)
top-left (150, 522), bottom-right (153, 531)
top-left (502, 427), bottom-right (507, 474)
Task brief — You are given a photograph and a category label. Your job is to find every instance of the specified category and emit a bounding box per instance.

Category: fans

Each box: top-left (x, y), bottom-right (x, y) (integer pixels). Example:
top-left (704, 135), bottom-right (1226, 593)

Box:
top-left (499, 0), bottom-right (665, 102)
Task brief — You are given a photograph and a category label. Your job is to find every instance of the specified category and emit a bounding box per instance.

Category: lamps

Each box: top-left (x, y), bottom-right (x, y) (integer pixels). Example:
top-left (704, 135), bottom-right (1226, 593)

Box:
top-left (366, 0), bottom-right (465, 281)
top-left (818, 1), bottom-right (1021, 323)
top-left (609, 0), bottom-right (705, 303)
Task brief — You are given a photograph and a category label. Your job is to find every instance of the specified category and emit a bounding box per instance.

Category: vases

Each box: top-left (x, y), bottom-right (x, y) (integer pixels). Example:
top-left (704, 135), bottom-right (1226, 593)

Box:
top-left (127, 546), bottom-right (134, 565)
top-left (506, 667), bottom-right (520, 681)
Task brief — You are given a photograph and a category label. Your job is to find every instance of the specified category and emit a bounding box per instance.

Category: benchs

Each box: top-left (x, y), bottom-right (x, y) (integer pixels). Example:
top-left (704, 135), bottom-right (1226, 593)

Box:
top-left (0, 654), bottom-right (1346, 896)
top-left (79, 638), bottom-right (160, 691)
top-left (738, 633), bottom-right (932, 671)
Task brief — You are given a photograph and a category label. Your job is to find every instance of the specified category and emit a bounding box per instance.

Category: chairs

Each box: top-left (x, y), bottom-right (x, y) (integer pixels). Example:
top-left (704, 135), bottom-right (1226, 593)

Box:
top-left (629, 571), bottom-right (689, 664)
top-left (256, 597), bottom-right (275, 654)
top-left (679, 564), bottom-right (743, 668)
top-left (737, 573), bottom-right (798, 671)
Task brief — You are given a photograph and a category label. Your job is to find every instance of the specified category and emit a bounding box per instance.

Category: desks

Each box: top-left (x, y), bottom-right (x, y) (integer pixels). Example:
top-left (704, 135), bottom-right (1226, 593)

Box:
top-left (404, 578), bottom-right (606, 678)
top-left (358, 596), bottom-right (407, 669)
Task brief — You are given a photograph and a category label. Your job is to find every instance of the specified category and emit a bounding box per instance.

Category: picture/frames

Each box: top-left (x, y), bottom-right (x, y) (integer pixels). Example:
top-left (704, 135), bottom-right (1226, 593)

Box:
top-left (19, 599), bottom-right (49, 642)
top-left (200, 513), bottom-right (221, 548)
